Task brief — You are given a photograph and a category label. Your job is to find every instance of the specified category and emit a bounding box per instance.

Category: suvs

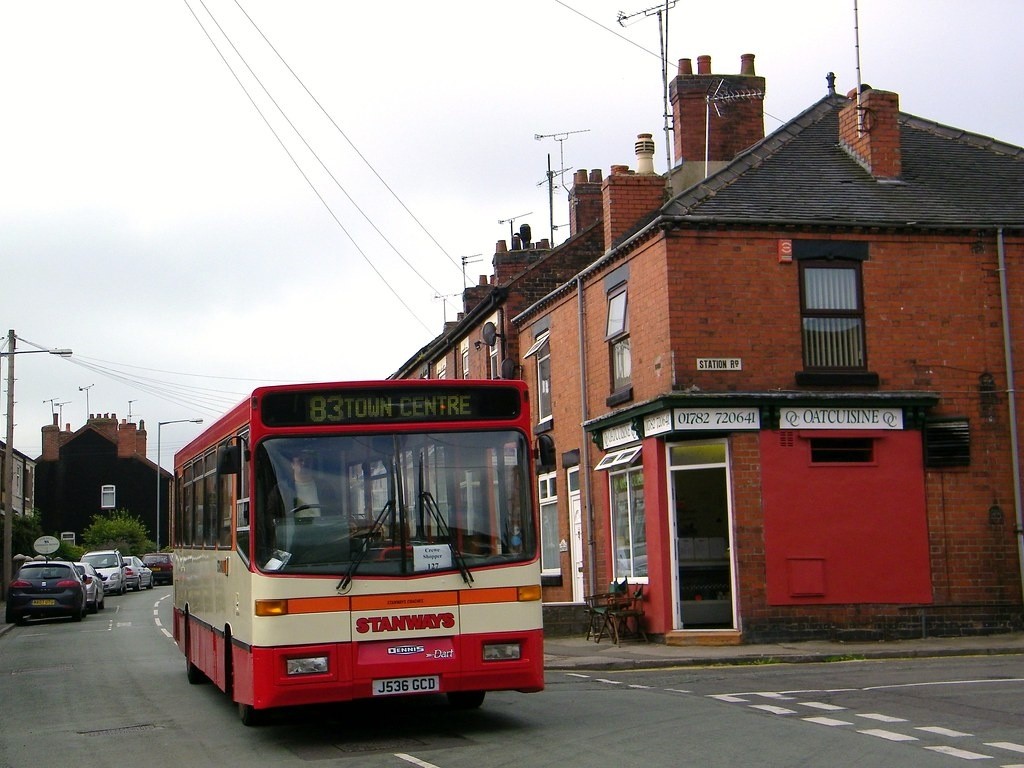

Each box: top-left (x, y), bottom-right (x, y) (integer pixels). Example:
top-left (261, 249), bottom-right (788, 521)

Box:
top-left (79, 548), bottom-right (128, 596)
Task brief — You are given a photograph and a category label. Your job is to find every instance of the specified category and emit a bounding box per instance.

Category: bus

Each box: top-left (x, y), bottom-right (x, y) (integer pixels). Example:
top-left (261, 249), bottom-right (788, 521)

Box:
top-left (171, 379), bottom-right (557, 728)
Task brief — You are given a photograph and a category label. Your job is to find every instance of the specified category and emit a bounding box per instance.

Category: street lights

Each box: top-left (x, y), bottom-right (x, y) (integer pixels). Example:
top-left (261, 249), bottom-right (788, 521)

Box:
top-left (157, 419), bottom-right (205, 551)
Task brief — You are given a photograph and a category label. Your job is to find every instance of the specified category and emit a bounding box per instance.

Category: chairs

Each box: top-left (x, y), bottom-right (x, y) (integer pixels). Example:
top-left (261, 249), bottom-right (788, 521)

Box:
top-left (584, 576), bottom-right (650, 649)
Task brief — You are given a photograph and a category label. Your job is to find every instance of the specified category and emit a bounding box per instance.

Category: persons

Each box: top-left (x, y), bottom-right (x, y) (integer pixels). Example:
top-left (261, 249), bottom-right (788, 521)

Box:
top-left (344, 519), bottom-right (386, 539)
top-left (266, 448), bottom-right (343, 528)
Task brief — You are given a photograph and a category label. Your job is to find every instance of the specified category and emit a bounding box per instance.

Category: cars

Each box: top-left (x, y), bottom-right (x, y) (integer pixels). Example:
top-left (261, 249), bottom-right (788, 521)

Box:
top-left (73, 561), bottom-right (105, 614)
top-left (122, 556), bottom-right (154, 591)
top-left (141, 553), bottom-right (173, 586)
top-left (5, 560), bottom-right (87, 625)
